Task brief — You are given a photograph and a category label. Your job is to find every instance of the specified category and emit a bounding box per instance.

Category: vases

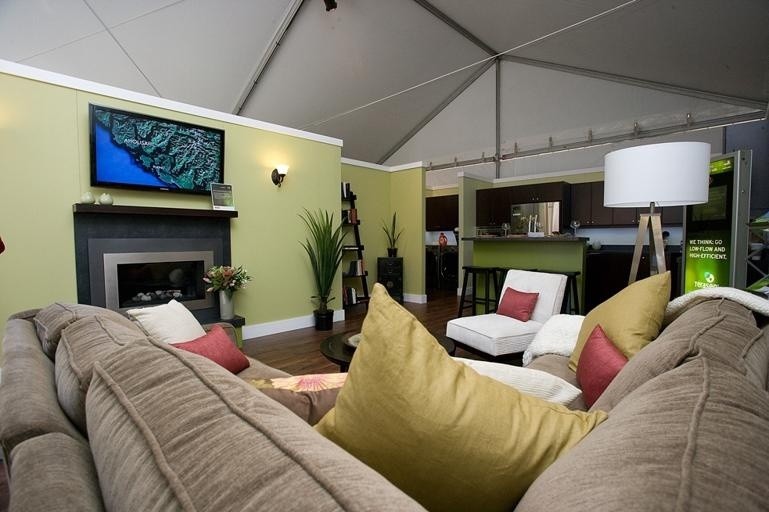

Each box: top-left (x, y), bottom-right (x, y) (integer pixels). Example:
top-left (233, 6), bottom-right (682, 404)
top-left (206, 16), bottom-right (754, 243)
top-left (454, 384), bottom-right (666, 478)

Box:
top-left (218, 288), bottom-right (234, 322)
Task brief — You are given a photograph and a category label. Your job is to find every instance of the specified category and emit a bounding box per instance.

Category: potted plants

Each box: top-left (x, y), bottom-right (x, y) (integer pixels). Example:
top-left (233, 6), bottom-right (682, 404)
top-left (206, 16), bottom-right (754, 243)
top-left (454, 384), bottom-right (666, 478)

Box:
top-left (292, 205), bottom-right (350, 331)
top-left (379, 209), bottom-right (407, 257)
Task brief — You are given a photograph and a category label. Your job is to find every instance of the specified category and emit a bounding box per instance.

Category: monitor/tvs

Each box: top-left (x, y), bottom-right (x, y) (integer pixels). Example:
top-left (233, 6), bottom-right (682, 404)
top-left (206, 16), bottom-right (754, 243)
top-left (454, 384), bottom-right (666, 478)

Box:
top-left (88, 104), bottom-right (225, 196)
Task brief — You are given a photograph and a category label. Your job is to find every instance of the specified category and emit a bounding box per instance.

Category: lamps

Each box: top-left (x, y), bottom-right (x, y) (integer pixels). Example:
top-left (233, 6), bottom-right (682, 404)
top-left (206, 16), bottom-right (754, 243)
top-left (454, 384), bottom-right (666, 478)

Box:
top-left (271, 163), bottom-right (290, 190)
top-left (602, 139), bottom-right (711, 287)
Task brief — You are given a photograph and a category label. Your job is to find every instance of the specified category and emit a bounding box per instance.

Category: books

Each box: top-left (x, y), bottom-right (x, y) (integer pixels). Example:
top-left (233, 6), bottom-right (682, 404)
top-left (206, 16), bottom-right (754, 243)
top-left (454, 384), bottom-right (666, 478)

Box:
top-left (341, 180), bottom-right (367, 306)
top-left (210, 182), bottom-right (235, 211)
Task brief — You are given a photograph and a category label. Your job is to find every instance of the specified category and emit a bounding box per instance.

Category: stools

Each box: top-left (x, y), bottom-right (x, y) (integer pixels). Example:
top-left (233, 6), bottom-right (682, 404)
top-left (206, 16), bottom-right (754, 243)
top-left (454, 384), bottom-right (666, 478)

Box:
top-left (455, 265), bottom-right (586, 319)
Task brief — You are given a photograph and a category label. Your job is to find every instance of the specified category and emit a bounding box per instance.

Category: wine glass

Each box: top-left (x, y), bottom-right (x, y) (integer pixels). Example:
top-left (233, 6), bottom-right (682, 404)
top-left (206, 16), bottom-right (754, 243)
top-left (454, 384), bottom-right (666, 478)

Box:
top-left (502, 222), bottom-right (510, 236)
top-left (571, 220), bottom-right (581, 237)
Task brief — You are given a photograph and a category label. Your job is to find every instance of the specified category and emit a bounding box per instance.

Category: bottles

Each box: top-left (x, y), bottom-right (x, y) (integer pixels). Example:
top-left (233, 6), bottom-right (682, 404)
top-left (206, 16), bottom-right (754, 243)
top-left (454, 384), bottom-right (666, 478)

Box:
top-left (439, 232), bottom-right (448, 247)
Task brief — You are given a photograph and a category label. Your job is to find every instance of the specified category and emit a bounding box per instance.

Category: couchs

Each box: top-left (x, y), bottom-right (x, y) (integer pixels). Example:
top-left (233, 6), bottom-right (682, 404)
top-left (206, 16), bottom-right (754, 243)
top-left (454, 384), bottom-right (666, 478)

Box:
top-left (0, 284), bottom-right (768, 510)
top-left (446, 267), bottom-right (569, 369)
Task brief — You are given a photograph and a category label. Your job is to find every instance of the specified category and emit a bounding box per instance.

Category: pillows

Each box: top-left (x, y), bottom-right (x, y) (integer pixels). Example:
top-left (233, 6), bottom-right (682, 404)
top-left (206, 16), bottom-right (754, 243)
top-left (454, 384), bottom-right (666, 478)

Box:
top-left (308, 280), bottom-right (609, 511)
top-left (162, 325), bottom-right (250, 376)
top-left (124, 296), bottom-right (208, 348)
top-left (562, 268), bottom-right (674, 411)
top-left (239, 364), bottom-right (348, 428)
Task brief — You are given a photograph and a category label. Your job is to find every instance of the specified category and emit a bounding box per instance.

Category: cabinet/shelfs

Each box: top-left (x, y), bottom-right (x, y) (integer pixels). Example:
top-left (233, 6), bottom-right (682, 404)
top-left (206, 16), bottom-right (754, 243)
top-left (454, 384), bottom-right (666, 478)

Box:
top-left (475, 181), bottom-right (510, 230)
top-left (568, 181), bottom-right (612, 228)
top-left (340, 191), bottom-right (371, 321)
top-left (612, 203), bottom-right (662, 227)
top-left (508, 180), bottom-right (572, 229)
top-left (662, 206), bottom-right (684, 226)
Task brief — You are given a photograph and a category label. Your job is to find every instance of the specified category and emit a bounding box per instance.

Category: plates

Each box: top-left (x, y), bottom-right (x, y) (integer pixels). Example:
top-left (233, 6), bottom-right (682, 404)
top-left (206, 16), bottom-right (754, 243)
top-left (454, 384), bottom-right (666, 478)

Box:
top-left (342, 330), bottom-right (361, 348)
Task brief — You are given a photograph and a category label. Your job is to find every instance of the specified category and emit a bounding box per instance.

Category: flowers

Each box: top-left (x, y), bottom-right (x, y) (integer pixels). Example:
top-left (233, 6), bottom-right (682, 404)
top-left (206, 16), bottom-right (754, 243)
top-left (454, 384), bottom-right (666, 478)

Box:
top-left (203, 263), bottom-right (252, 303)
